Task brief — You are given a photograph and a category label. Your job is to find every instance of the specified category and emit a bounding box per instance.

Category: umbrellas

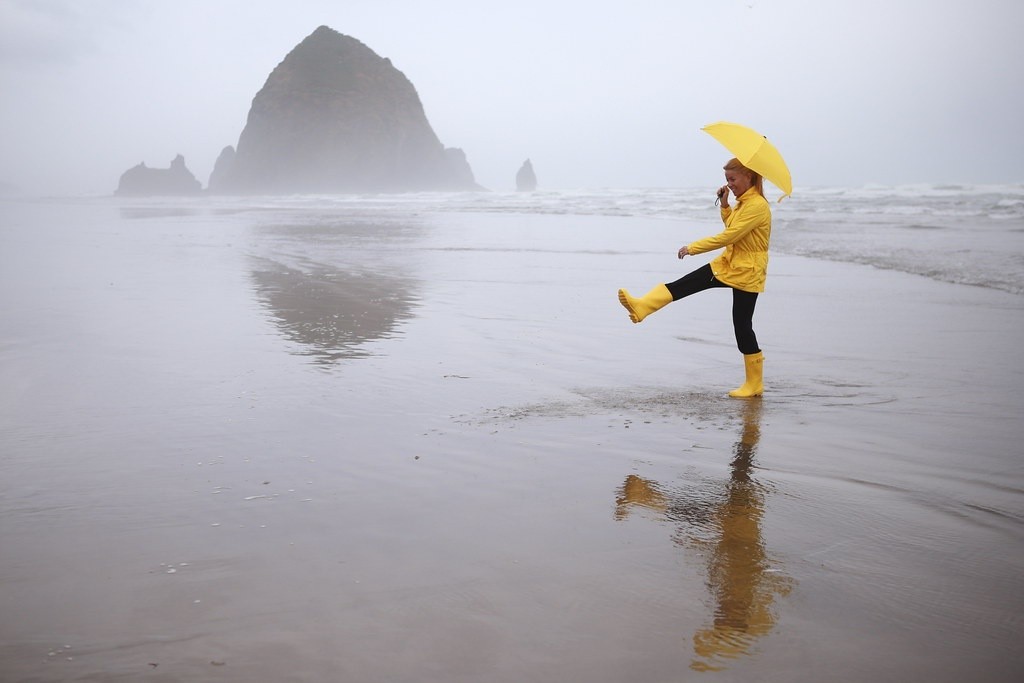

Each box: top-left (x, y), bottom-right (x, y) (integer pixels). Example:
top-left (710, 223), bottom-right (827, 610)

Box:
top-left (699, 121), bottom-right (794, 206)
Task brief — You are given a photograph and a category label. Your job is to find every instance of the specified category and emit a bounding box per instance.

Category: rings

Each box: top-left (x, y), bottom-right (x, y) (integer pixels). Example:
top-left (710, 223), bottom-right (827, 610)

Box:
top-left (679, 251), bottom-right (683, 256)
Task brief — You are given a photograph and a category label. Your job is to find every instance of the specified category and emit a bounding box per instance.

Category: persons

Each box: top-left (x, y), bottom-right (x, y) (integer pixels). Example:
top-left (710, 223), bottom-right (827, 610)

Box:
top-left (618, 157), bottom-right (772, 398)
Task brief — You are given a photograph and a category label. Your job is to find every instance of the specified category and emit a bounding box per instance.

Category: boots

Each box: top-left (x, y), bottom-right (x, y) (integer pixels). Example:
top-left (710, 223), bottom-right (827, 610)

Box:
top-left (619, 284), bottom-right (674, 323)
top-left (729, 348), bottom-right (764, 398)
